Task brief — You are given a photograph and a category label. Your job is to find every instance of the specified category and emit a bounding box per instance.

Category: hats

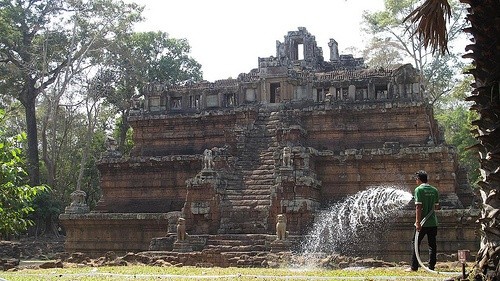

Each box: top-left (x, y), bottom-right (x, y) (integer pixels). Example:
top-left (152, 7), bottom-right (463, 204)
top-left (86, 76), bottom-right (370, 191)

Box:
top-left (415, 170), bottom-right (428, 179)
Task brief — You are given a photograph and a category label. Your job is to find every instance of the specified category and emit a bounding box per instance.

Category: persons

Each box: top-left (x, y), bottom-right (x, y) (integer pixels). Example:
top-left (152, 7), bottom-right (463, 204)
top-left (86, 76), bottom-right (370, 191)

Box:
top-left (404, 170), bottom-right (439, 273)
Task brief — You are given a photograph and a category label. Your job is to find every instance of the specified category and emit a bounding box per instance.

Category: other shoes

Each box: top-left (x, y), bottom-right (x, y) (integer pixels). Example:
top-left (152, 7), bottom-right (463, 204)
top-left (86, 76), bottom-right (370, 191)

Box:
top-left (426, 266), bottom-right (434, 271)
top-left (404, 266), bottom-right (417, 272)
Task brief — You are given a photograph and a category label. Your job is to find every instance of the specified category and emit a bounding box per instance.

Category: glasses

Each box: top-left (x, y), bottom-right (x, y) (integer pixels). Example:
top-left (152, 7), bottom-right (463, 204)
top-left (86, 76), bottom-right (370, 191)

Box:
top-left (416, 177), bottom-right (420, 179)
